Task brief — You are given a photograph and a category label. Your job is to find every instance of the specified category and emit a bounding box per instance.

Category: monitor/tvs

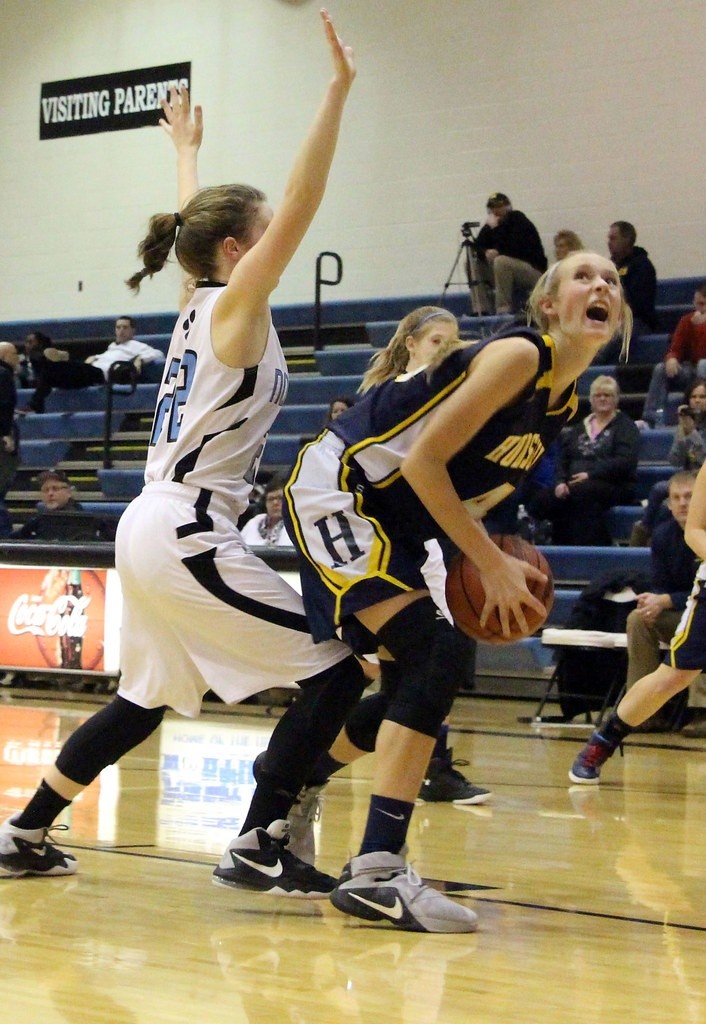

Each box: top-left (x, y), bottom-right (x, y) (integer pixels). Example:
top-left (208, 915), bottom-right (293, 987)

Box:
top-left (43, 510), bottom-right (115, 542)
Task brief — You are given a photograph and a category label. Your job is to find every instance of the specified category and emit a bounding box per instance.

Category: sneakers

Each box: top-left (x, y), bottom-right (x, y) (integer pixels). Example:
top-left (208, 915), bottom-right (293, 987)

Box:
top-left (569, 728), bottom-right (618, 785)
top-left (252, 749), bottom-right (330, 867)
top-left (417, 748), bottom-right (492, 805)
top-left (213, 820), bottom-right (341, 900)
top-left (0, 811), bottom-right (79, 877)
top-left (330, 841), bottom-right (478, 932)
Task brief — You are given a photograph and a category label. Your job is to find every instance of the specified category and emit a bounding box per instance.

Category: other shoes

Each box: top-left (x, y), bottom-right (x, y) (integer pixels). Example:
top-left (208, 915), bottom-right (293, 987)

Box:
top-left (635, 417), bottom-right (656, 432)
top-left (14, 404), bottom-right (36, 415)
top-left (43, 348), bottom-right (69, 362)
top-left (681, 715), bottom-right (706, 738)
top-left (462, 311), bottom-right (491, 317)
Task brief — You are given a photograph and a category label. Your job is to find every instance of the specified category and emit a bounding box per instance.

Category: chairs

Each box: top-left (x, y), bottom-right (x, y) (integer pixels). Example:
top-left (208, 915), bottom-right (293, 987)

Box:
top-left (515, 566), bottom-right (695, 734)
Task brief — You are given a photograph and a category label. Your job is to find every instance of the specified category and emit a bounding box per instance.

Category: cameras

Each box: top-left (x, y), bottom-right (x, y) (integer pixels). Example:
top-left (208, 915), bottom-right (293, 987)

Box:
top-left (462, 222), bottom-right (479, 229)
top-left (681, 408), bottom-right (702, 416)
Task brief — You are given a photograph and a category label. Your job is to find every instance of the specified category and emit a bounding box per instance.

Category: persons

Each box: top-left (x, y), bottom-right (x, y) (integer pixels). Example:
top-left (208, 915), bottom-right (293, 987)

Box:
top-left (240, 480), bottom-right (295, 547)
top-left (330, 396), bottom-right (354, 421)
top-left (591, 221), bottom-right (657, 366)
top-left (359, 306), bottom-right (492, 806)
top-left (517, 428), bottom-right (560, 498)
top-left (464, 193), bottom-right (548, 316)
top-left (0, 331), bottom-right (53, 539)
top-left (500, 230), bottom-right (582, 330)
top-left (634, 288), bottom-right (706, 430)
top-left (626, 468), bottom-right (706, 738)
top-left (0, 6), bottom-right (373, 899)
top-left (540, 375), bottom-right (639, 547)
top-left (569, 457), bottom-right (706, 784)
top-left (14, 316), bottom-right (165, 415)
top-left (253, 250), bottom-right (633, 933)
top-left (628, 379), bottom-right (706, 547)
top-left (10, 469), bottom-right (110, 543)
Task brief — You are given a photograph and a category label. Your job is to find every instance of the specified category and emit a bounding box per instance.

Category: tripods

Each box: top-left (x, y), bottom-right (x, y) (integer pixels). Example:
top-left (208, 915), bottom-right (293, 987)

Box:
top-left (440, 229), bottom-right (492, 317)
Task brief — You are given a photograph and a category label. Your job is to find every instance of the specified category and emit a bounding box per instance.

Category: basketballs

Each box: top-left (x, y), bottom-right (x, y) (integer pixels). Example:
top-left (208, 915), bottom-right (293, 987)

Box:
top-left (444, 532), bottom-right (557, 649)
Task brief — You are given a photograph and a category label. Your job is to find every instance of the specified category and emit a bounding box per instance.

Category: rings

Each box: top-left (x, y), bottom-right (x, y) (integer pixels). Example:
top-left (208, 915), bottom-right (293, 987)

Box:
top-left (647, 611), bottom-right (652, 617)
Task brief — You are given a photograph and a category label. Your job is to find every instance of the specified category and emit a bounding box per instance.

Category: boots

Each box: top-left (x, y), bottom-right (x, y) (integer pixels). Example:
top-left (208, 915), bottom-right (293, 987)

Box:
top-left (628, 521), bottom-right (652, 548)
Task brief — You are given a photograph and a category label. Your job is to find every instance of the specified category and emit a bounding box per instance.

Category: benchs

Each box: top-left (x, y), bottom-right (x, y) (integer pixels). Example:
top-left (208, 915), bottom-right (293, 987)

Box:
top-left (0, 276), bottom-right (706, 703)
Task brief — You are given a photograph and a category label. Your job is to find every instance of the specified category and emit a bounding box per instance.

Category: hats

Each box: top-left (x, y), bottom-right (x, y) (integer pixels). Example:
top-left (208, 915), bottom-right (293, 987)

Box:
top-left (486, 193), bottom-right (511, 206)
top-left (36, 470), bottom-right (71, 485)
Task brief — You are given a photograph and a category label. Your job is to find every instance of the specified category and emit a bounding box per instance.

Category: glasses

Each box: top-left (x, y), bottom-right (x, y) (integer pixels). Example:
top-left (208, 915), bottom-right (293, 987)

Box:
top-left (42, 485), bottom-right (70, 493)
top-left (489, 203), bottom-right (504, 209)
